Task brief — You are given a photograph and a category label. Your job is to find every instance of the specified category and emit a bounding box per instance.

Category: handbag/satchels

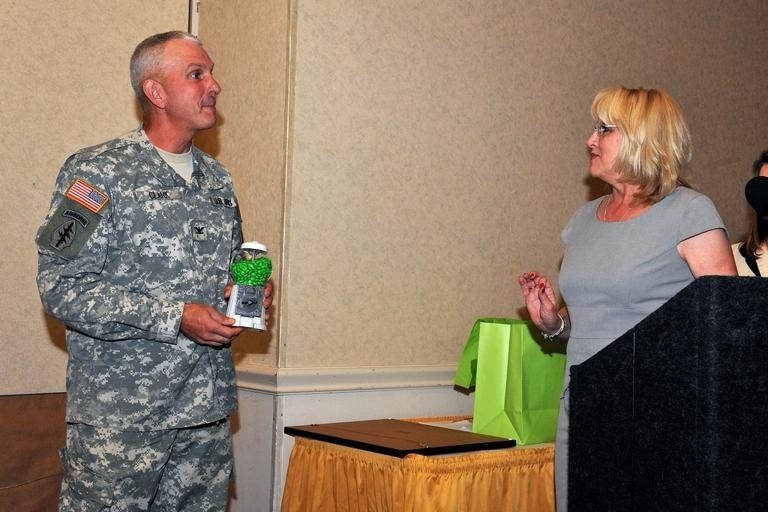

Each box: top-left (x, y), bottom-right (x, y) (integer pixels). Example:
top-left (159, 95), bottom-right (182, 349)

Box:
top-left (472, 320), bottom-right (568, 446)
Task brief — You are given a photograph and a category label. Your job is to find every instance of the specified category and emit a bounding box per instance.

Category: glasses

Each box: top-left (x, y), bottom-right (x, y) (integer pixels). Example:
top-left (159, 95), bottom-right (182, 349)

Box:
top-left (593, 124), bottom-right (619, 137)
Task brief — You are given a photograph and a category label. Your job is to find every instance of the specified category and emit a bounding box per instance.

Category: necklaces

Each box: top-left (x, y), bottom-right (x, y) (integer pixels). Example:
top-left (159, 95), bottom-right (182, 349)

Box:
top-left (602, 196), bottom-right (632, 222)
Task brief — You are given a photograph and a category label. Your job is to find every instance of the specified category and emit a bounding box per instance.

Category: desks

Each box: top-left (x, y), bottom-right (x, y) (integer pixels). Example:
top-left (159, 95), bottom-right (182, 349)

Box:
top-left (273, 410), bottom-right (559, 512)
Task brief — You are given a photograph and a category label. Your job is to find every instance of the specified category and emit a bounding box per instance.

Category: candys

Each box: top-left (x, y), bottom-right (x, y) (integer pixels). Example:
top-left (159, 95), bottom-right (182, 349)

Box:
top-left (231, 257), bottom-right (272, 286)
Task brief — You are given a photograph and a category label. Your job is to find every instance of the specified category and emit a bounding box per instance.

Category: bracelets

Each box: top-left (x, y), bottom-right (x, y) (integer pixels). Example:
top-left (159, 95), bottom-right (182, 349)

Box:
top-left (540, 312), bottom-right (565, 341)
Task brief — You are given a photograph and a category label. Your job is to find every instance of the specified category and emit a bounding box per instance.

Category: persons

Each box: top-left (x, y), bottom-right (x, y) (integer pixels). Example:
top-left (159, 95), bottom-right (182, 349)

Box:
top-left (35, 30), bottom-right (274, 512)
top-left (517, 83), bottom-right (738, 511)
top-left (729, 149), bottom-right (768, 277)
